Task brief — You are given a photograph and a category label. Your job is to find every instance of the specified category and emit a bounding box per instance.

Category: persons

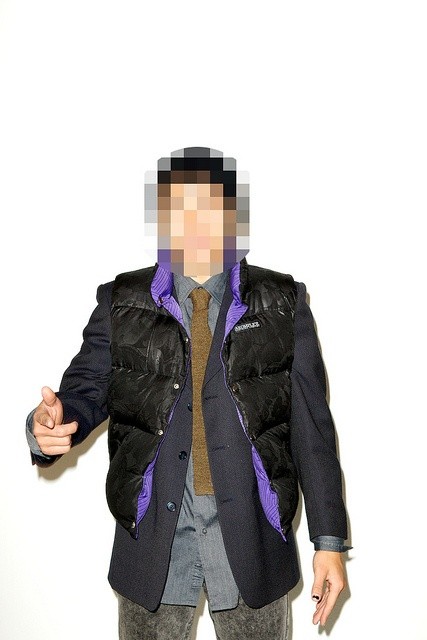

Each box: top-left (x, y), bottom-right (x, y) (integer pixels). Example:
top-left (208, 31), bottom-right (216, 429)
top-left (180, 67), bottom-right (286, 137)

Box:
top-left (26, 148), bottom-right (354, 640)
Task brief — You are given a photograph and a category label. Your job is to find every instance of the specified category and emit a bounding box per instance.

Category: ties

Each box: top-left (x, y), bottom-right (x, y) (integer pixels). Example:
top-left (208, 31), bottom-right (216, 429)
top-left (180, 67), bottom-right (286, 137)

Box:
top-left (187, 288), bottom-right (216, 496)
